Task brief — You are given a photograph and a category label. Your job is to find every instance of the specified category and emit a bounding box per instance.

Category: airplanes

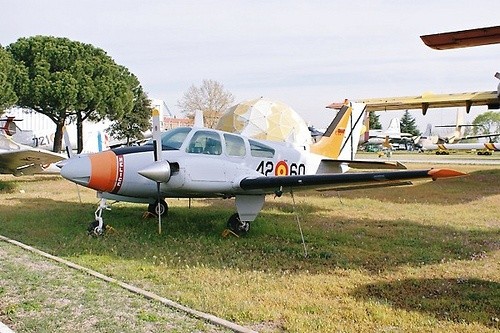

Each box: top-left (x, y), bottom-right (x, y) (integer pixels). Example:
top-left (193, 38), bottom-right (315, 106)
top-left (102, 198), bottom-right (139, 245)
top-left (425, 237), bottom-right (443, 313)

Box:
top-left (55, 104), bottom-right (466, 257)
top-left (0, 114), bottom-right (66, 177)
top-left (309, 25), bottom-right (500, 158)
